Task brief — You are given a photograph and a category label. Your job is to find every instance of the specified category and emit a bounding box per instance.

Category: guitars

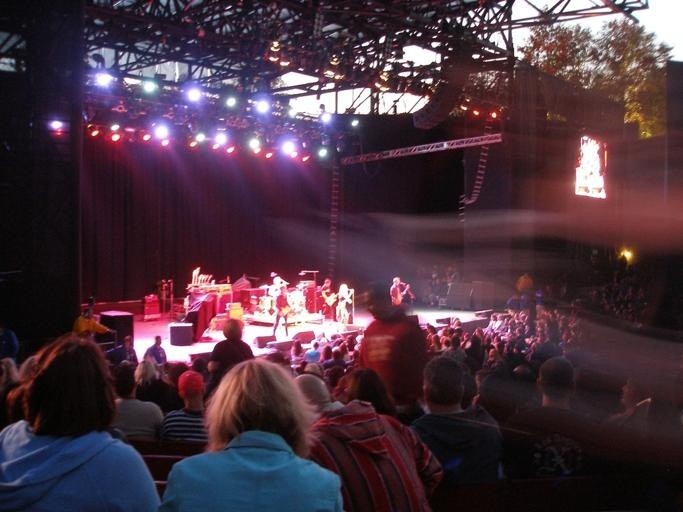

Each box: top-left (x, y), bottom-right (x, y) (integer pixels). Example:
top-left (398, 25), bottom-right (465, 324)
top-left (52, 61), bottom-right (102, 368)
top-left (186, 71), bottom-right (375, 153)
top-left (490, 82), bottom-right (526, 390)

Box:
top-left (393, 282), bottom-right (409, 305)
top-left (326, 292), bottom-right (339, 307)
top-left (281, 302), bottom-right (294, 316)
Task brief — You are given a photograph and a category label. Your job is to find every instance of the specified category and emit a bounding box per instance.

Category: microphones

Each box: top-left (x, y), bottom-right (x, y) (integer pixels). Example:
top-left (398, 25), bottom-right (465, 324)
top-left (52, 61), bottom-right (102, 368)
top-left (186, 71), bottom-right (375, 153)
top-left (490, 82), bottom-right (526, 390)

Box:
top-left (400, 282), bottom-right (406, 284)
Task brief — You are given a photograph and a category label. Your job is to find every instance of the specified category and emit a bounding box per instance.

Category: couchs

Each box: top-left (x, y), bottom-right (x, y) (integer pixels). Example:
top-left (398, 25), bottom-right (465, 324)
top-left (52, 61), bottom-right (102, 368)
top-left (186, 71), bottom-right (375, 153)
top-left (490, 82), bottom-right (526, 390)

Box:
top-left (439, 282), bottom-right (476, 310)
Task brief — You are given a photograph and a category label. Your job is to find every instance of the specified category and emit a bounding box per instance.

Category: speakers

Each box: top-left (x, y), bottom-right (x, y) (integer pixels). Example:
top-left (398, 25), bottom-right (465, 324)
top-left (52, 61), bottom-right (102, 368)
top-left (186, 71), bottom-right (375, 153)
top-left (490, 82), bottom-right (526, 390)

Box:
top-left (331, 331), bottom-right (358, 340)
top-left (189, 352), bottom-right (213, 365)
top-left (475, 310), bottom-right (494, 317)
top-left (254, 336), bottom-right (276, 348)
top-left (436, 317), bottom-right (454, 324)
top-left (267, 340), bottom-right (295, 352)
top-left (292, 331), bottom-right (316, 344)
top-left (413, 39), bottom-right (472, 130)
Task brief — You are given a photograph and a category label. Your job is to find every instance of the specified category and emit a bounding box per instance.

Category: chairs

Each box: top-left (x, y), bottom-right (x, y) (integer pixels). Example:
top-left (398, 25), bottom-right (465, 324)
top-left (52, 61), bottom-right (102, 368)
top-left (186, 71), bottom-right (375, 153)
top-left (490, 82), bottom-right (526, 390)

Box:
top-left (615, 397), bottom-right (652, 452)
top-left (438, 472), bottom-right (581, 509)
top-left (128, 435), bottom-right (207, 498)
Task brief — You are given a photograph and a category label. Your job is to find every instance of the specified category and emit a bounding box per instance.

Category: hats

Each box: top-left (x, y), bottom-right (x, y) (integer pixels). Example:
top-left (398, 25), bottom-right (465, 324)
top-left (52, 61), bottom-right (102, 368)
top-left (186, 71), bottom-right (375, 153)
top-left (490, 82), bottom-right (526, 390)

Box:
top-left (177, 370), bottom-right (204, 398)
